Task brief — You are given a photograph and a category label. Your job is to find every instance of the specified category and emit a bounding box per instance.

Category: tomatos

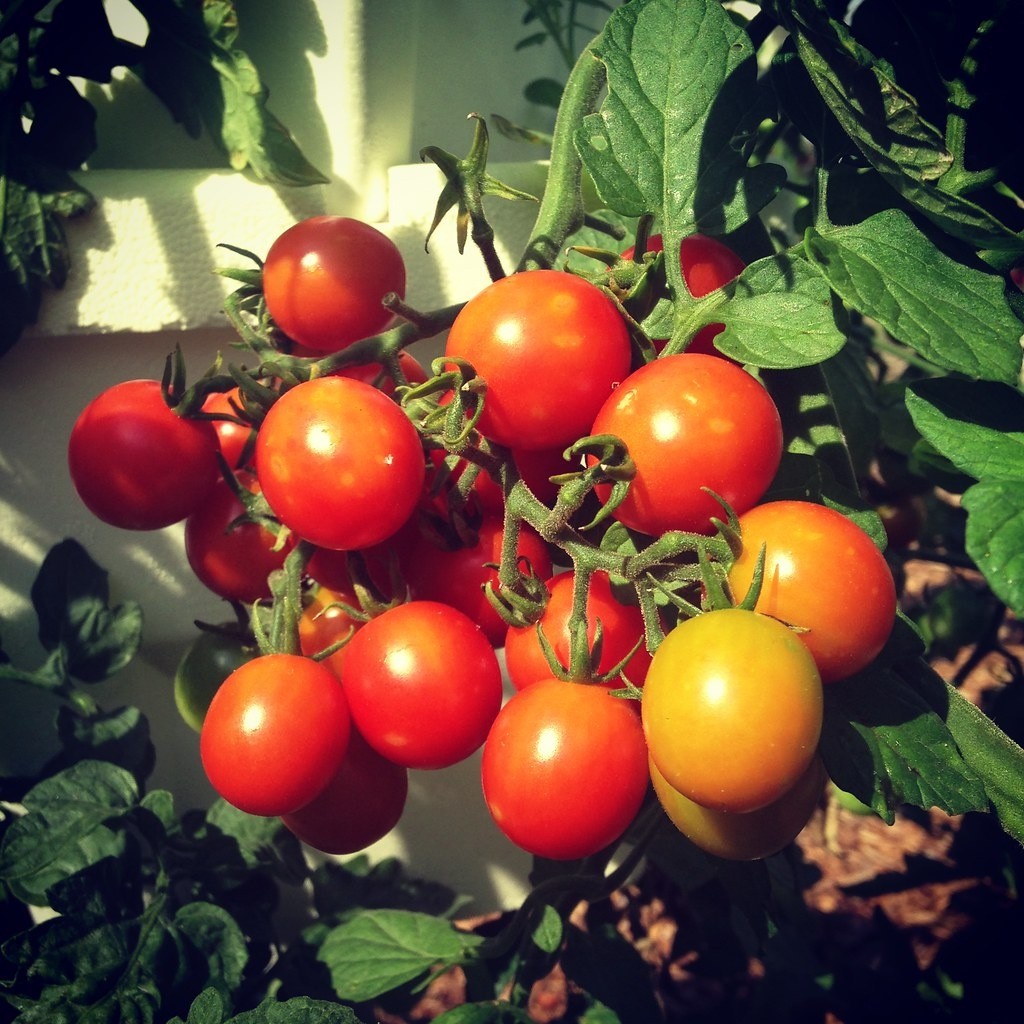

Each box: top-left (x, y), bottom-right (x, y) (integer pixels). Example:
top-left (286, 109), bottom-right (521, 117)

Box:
top-left (68, 215), bottom-right (897, 864)
